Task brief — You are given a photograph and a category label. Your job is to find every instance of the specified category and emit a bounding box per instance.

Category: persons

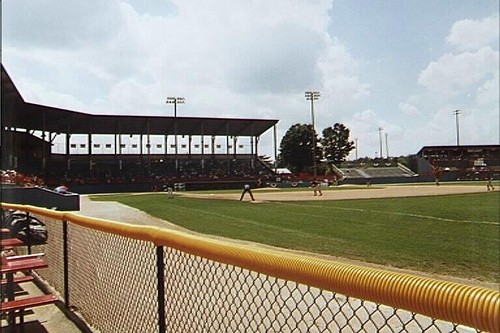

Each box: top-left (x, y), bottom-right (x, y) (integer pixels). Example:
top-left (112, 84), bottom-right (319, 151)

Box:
top-left (45, 165), bottom-right (119, 184)
top-left (225, 157), bottom-right (272, 178)
top-left (167, 179), bottom-right (174, 198)
top-left (311, 177), bottom-right (323, 196)
top-left (123, 158), bottom-right (227, 180)
top-left (0, 169), bottom-right (44, 188)
top-left (239, 184), bottom-right (255, 201)
top-left (484, 172), bottom-right (494, 190)
top-left (435, 174), bottom-right (439, 185)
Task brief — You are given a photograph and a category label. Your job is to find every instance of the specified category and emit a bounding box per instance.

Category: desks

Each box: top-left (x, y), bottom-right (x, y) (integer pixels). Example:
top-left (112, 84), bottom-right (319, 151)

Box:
top-left (0, 255), bottom-right (49, 328)
top-left (0, 239), bottom-right (24, 252)
top-left (0, 226), bottom-right (11, 233)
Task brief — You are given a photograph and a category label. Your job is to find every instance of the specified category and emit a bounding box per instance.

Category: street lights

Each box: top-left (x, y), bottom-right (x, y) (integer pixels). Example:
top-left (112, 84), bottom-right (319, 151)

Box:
top-left (165, 96), bottom-right (186, 172)
top-left (452, 109), bottom-right (461, 146)
top-left (305, 91), bottom-right (321, 180)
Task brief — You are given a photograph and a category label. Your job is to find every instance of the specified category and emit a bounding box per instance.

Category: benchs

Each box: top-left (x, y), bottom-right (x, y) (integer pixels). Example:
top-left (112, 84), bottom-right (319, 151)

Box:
top-left (0, 274), bottom-right (60, 313)
